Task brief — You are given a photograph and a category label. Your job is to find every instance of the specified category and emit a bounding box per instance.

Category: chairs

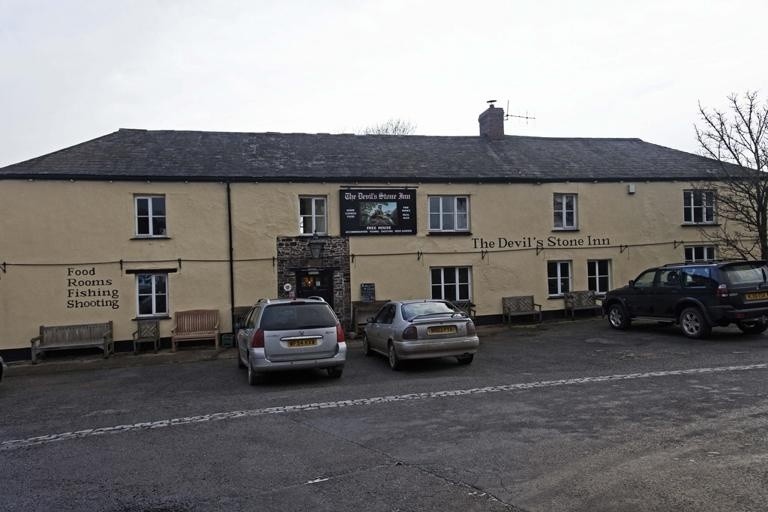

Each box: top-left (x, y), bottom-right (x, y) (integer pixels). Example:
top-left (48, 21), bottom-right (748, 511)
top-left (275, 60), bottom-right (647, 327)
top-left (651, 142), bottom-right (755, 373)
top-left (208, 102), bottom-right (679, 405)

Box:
top-left (132, 320), bottom-right (160, 355)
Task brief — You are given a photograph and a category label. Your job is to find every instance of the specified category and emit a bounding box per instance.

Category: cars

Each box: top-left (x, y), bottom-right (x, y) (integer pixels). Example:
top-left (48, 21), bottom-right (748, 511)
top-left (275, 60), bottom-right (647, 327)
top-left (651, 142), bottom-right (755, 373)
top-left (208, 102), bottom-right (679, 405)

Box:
top-left (602, 257), bottom-right (768, 339)
top-left (236, 295), bottom-right (480, 385)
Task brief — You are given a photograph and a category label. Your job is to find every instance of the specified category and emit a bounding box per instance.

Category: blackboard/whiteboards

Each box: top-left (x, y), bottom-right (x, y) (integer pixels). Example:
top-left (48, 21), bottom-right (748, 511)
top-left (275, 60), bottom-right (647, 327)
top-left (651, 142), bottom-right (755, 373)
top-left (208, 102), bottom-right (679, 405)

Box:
top-left (360, 283), bottom-right (375, 304)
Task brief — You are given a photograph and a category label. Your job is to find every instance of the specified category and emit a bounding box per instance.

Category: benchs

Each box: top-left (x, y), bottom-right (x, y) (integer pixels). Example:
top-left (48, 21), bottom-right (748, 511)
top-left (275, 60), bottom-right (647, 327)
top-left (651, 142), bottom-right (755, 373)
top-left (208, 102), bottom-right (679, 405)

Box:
top-left (31, 320), bottom-right (114, 364)
top-left (502, 295), bottom-right (542, 328)
top-left (234, 306), bottom-right (251, 348)
top-left (353, 300), bottom-right (392, 335)
top-left (451, 301), bottom-right (476, 327)
top-left (564, 290), bottom-right (604, 321)
top-left (171, 309), bottom-right (220, 354)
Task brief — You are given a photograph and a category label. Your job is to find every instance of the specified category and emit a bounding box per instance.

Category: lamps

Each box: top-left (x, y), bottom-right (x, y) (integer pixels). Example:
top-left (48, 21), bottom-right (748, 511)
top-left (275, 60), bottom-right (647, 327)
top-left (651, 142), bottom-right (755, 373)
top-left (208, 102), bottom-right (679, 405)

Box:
top-left (308, 230), bottom-right (326, 259)
